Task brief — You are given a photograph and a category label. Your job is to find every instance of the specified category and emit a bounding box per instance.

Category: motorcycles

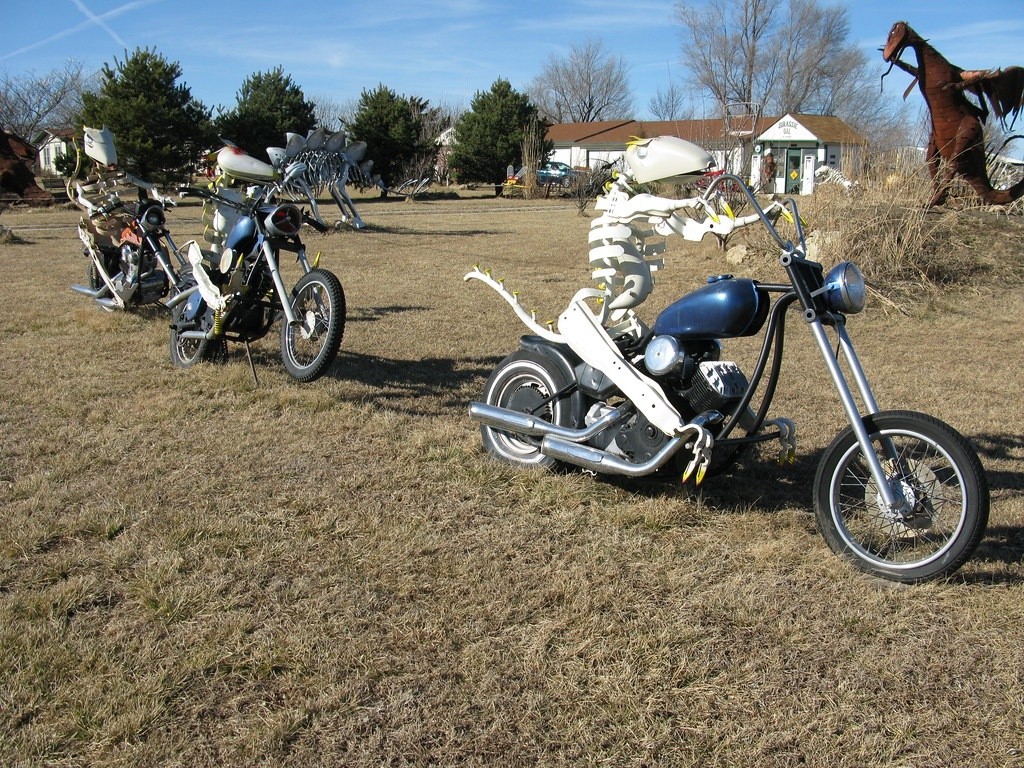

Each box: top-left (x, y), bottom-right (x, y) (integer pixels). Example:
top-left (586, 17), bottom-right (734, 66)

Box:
top-left (466, 174), bottom-right (992, 585)
top-left (68, 185), bottom-right (346, 384)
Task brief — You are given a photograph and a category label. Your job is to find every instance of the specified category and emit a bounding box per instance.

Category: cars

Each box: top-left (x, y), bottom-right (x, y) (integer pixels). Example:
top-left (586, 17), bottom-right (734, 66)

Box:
top-left (535, 160), bottom-right (575, 188)
